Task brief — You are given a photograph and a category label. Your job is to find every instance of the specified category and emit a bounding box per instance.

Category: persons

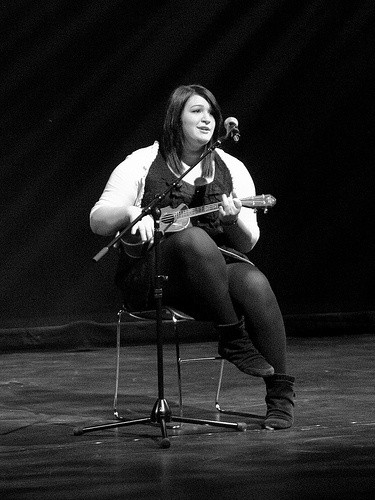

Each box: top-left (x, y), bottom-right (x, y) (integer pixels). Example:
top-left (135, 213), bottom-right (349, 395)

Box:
top-left (90, 85), bottom-right (295, 430)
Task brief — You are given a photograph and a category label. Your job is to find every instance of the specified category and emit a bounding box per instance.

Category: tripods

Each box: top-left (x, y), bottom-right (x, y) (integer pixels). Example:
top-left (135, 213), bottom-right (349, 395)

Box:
top-left (73, 137), bottom-right (248, 449)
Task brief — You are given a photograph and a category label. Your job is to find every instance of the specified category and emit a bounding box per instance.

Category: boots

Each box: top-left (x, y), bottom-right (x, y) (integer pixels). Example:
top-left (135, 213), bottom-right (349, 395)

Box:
top-left (261, 372), bottom-right (296, 429)
top-left (216, 314), bottom-right (275, 376)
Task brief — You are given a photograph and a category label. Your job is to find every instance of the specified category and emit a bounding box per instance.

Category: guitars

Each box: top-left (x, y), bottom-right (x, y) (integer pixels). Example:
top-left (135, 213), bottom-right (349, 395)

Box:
top-left (119, 190), bottom-right (277, 258)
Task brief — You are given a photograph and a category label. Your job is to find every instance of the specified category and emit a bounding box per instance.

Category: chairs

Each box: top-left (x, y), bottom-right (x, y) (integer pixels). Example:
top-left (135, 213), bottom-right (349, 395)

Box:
top-left (112, 304), bottom-right (266, 430)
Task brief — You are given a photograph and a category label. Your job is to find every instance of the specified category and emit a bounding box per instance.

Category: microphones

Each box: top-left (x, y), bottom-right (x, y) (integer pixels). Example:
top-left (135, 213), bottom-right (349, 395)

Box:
top-left (223, 116), bottom-right (239, 143)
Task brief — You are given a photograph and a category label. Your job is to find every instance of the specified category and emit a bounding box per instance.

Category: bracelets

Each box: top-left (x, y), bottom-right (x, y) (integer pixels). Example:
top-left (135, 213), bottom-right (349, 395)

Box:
top-left (219, 218), bottom-right (239, 226)
